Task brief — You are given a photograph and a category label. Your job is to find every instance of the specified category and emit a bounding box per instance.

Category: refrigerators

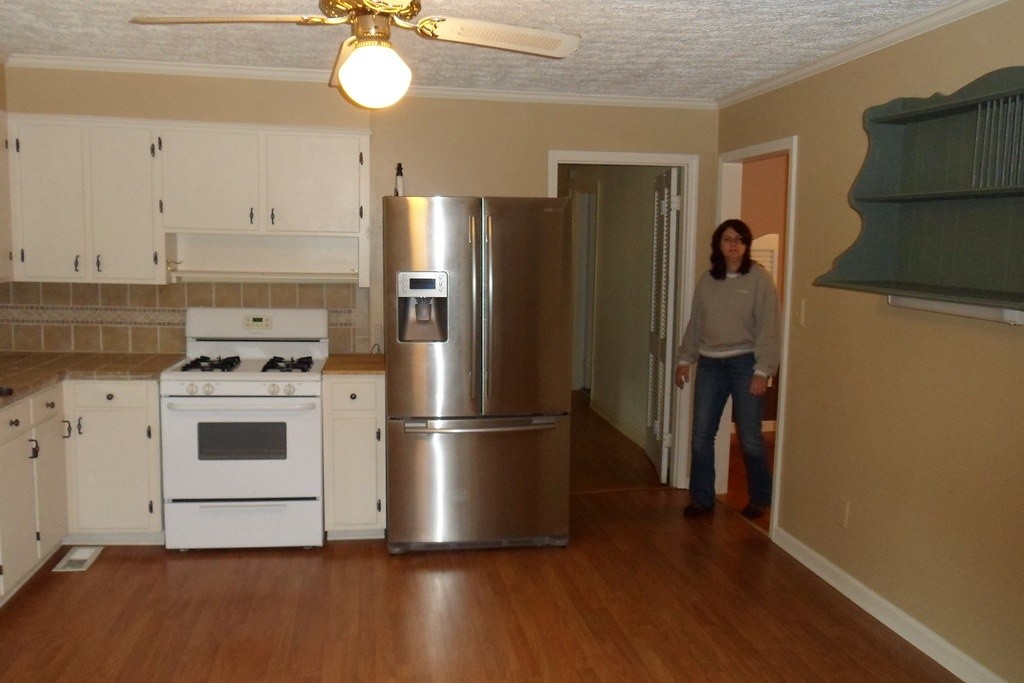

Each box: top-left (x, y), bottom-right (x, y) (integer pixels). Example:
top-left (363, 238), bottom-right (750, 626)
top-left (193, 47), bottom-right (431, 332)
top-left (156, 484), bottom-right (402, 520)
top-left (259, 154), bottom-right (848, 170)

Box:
top-left (382, 197), bottom-right (571, 556)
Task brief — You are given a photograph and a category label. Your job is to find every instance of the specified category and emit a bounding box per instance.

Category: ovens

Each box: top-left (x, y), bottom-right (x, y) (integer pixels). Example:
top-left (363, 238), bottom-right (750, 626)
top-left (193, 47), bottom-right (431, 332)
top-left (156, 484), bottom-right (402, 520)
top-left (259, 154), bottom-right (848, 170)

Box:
top-left (158, 381), bottom-right (323, 549)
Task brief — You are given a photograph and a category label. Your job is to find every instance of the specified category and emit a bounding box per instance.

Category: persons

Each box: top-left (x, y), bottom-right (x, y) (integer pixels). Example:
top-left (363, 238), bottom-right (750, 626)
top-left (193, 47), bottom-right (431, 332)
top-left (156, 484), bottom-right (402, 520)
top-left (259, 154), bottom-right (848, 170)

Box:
top-left (674, 218), bottom-right (782, 518)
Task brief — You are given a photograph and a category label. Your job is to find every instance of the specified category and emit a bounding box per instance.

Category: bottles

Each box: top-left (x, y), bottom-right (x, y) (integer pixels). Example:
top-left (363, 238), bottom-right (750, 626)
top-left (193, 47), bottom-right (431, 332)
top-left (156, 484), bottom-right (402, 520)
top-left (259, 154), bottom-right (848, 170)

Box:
top-left (393, 163), bottom-right (404, 197)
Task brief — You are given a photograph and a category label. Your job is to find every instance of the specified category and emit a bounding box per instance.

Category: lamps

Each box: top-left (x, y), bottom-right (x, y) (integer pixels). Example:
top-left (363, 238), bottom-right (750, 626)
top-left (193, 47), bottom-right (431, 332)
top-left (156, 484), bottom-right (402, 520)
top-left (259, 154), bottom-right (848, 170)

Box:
top-left (337, 14), bottom-right (412, 109)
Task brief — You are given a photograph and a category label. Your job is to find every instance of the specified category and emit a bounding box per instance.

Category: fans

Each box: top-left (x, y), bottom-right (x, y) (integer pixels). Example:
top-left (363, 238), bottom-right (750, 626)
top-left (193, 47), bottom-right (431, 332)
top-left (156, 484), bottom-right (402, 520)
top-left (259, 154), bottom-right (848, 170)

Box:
top-left (130, 0), bottom-right (583, 87)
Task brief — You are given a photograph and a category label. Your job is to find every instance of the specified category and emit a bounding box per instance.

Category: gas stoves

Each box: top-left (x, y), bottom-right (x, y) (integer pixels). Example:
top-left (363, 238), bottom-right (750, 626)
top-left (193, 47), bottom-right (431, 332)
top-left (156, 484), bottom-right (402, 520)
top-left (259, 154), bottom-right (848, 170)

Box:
top-left (158, 306), bottom-right (330, 378)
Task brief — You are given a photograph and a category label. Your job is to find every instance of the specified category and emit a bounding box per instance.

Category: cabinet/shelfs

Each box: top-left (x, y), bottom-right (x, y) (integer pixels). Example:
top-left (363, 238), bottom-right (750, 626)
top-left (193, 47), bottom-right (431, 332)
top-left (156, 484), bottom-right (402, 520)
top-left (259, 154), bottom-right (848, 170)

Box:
top-left (15, 122), bottom-right (158, 280)
top-left (158, 128), bottom-right (364, 233)
top-left (322, 374), bottom-right (387, 532)
top-left (811, 64), bottom-right (1024, 313)
top-left (0, 385), bottom-right (72, 594)
top-left (74, 384), bottom-right (154, 531)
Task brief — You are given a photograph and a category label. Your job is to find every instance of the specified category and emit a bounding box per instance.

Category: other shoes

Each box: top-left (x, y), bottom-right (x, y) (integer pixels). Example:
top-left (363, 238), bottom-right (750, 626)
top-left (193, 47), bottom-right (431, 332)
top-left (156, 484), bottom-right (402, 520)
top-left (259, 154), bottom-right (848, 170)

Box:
top-left (685, 500), bottom-right (708, 517)
top-left (740, 502), bottom-right (769, 520)
